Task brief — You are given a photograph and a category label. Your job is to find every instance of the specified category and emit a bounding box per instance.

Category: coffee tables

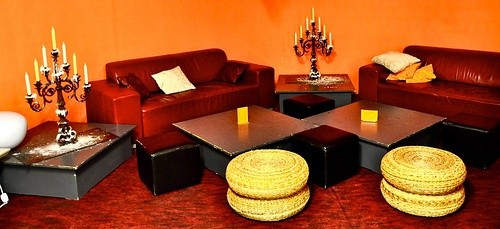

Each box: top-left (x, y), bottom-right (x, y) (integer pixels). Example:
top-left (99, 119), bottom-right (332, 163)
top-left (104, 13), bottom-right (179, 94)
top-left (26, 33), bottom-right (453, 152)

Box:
top-left (302, 100), bottom-right (447, 174)
top-left (172, 104), bottom-right (320, 179)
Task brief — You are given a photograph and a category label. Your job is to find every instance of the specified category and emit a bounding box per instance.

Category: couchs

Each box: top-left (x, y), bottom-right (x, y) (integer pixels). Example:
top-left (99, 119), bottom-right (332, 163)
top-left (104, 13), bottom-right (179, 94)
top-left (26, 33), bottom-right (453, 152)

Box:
top-left (360, 45), bottom-right (500, 169)
top-left (85, 48), bottom-right (274, 153)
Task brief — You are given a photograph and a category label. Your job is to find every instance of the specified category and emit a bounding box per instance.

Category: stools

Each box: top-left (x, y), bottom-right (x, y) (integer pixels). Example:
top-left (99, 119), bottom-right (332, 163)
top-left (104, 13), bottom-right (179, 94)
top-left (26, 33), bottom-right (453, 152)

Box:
top-left (225, 149), bottom-right (311, 222)
top-left (381, 145), bottom-right (467, 217)
top-left (292, 125), bottom-right (359, 190)
top-left (136, 130), bottom-right (202, 196)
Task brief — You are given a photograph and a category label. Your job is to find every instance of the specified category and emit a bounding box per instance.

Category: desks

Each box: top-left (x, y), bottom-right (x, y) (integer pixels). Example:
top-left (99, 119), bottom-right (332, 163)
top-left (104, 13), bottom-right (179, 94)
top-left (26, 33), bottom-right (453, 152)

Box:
top-left (275, 74), bottom-right (355, 113)
top-left (0, 121), bottom-right (136, 201)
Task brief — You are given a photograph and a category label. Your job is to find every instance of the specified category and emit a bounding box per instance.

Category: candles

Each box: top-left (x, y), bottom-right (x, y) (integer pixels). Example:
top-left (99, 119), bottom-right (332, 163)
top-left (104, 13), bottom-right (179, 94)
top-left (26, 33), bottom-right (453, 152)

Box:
top-left (83, 61), bottom-right (88, 84)
top-left (25, 72), bottom-right (31, 95)
top-left (42, 45), bottom-right (47, 67)
top-left (72, 52), bottom-right (77, 74)
top-left (34, 58), bottom-right (40, 81)
top-left (294, 7), bottom-right (332, 46)
top-left (62, 41), bottom-right (67, 63)
top-left (50, 27), bottom-right (57, 50)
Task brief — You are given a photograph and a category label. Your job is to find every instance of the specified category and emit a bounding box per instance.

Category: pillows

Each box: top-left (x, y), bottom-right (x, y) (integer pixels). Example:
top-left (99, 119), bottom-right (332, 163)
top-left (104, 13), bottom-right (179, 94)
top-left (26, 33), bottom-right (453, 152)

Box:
top-left (116, 62), bottom-right (250, 99)
top-left (371, 51), bottom-right (436, 83)
